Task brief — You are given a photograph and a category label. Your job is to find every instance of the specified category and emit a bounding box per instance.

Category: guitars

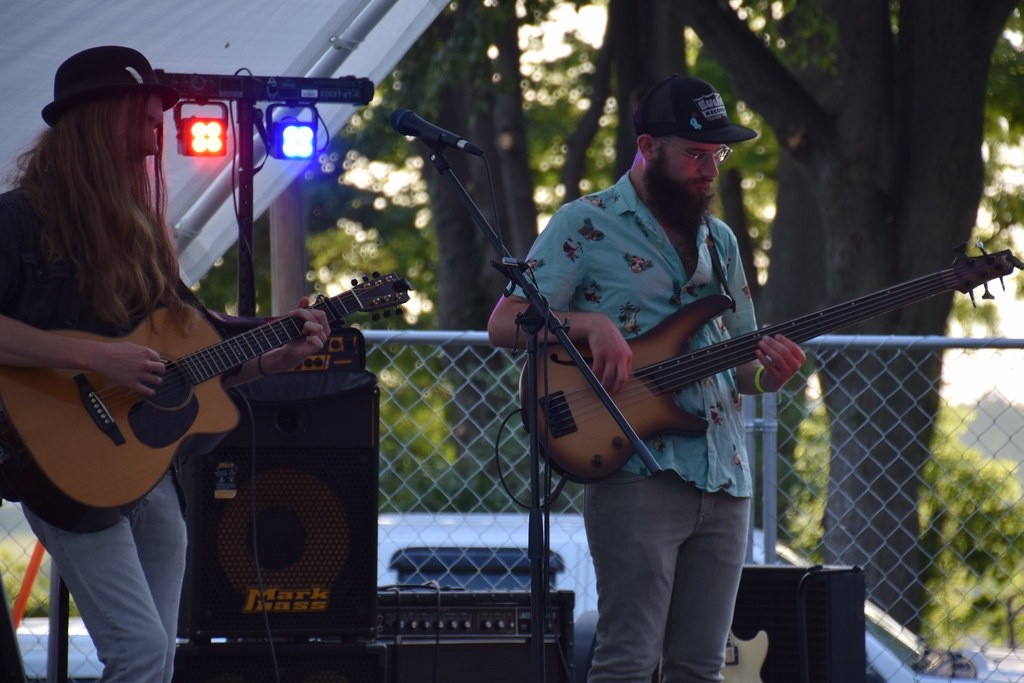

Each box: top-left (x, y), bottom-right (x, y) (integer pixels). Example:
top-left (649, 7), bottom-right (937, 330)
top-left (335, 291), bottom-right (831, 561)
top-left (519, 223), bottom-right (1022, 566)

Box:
top-left (0, 271), bottom-right (416, 533)
top-left (520, 243), bottom-right (1023, 486)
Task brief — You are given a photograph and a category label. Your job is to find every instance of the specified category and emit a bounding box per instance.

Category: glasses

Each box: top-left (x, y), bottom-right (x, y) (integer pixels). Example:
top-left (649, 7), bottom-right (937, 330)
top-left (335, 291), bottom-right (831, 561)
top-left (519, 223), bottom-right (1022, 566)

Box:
top-left (656, 136), bottom-right (732, 165)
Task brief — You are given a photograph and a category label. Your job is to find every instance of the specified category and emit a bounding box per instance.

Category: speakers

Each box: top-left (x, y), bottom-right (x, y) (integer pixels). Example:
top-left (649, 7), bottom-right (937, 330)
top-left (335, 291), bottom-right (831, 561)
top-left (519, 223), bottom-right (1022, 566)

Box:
top-left (730, 563), bottom-right (865, 683)
top-left (174, 326), bottom-right (571, 683)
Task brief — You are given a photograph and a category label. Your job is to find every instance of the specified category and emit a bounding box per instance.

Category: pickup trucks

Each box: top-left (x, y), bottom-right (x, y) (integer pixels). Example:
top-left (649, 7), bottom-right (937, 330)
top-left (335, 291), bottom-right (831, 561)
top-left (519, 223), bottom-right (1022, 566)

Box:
top-left (12, 507), bottom-right (1023, 683)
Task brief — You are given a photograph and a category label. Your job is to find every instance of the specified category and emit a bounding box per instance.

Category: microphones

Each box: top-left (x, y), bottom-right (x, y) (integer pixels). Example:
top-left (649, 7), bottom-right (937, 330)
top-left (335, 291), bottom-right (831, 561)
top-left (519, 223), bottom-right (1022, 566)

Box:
top-left (390, 108), bottom-right (484, 156)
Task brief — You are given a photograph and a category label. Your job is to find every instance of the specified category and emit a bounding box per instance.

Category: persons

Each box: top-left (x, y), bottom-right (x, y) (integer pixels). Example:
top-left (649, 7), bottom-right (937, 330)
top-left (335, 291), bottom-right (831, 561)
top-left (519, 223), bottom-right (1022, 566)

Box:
top-left (1, 45), bottom-right (330, 682)
top-left (488, 74), bottom-right (807, 682)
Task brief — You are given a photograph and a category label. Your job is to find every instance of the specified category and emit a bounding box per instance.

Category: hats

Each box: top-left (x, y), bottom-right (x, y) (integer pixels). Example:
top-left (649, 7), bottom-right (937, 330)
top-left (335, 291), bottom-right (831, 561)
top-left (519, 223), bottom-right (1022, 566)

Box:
top-left (42, 46), bottom-right (179, 125)
top-left (634, 71), bottom-right (758, 144)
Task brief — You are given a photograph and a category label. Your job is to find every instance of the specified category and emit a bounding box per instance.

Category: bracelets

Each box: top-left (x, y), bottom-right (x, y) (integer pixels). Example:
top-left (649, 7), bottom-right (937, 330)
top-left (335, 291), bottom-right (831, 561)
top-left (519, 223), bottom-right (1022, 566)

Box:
top-left (755, 364), bottom-right (768, 394)
top-left (258, 355), bottom-right (269, 376)
